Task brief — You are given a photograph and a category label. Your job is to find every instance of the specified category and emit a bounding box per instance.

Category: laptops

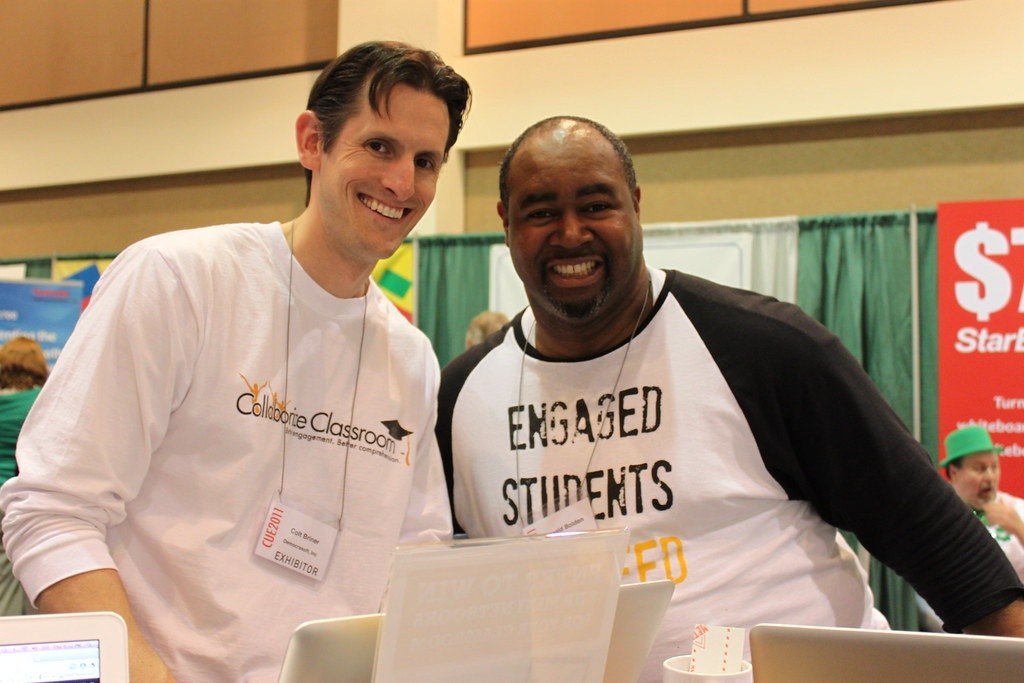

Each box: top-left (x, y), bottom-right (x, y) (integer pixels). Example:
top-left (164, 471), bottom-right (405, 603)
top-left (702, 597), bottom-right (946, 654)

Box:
top-left (749, 623), bottom-right (1024, 683)
top-left (277, 580), bottom-right (677, 683)
top-left (0, 611), bottom-right (129, 683)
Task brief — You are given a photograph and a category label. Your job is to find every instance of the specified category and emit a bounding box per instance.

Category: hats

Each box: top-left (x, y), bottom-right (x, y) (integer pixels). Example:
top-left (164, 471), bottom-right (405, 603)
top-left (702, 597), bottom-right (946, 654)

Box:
top-left (938, 425), bottom-right (1004, 466)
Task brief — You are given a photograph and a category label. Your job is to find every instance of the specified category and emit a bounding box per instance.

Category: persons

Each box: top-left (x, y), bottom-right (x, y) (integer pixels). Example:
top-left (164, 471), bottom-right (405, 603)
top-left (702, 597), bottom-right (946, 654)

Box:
top-left (431, 117), bottom-right (1023, 683)
top-left (0, 337), bottom-right (51, 618)
top-left (0, 37), bottom-right (456, 682)
top-left (909, 426), bottom-right (1023, 636)
